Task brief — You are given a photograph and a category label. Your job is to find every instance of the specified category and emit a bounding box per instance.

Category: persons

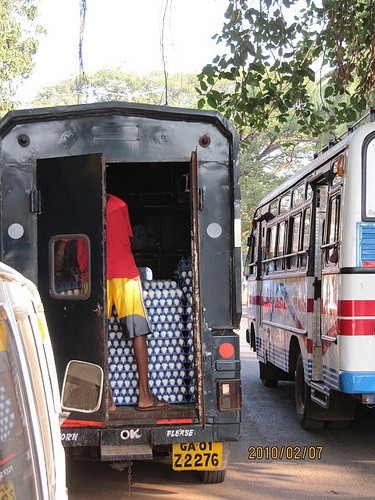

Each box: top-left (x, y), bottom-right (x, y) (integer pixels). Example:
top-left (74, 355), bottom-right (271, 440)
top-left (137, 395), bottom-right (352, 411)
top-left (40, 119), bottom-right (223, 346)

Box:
top-left (54, 192), bottom-right (172, 412)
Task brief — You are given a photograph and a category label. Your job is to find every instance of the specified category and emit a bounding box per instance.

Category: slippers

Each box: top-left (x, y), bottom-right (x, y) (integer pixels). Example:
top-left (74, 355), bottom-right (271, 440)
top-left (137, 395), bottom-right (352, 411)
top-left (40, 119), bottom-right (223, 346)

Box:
top-left (136, 394), bottom-right (172, 410)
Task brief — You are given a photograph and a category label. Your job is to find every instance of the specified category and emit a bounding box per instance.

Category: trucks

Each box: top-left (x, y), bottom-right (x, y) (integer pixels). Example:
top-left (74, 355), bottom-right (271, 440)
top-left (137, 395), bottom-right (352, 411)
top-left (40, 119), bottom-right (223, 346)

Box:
top-left (0, 102), bottom-right (242, 484)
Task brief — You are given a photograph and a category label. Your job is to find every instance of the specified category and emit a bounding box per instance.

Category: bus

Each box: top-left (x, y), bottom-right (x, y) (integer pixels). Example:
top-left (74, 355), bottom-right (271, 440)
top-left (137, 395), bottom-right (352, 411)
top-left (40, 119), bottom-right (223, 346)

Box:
top-left (243, 122), bottom-right (375, 428)
